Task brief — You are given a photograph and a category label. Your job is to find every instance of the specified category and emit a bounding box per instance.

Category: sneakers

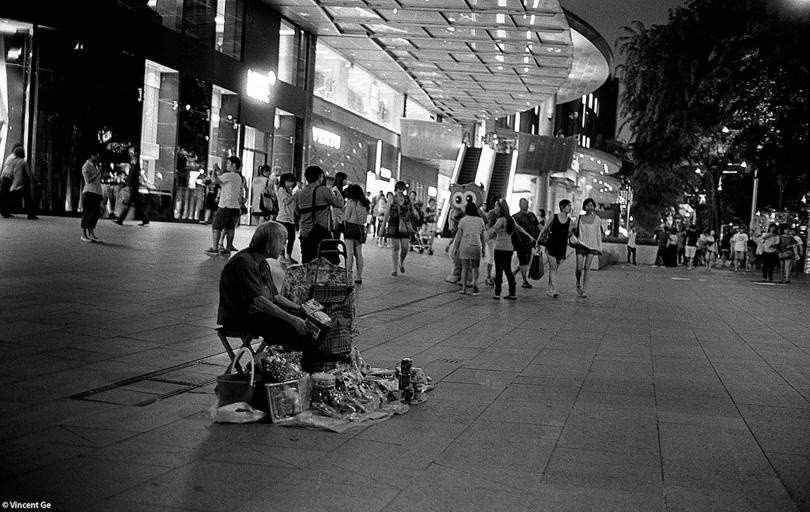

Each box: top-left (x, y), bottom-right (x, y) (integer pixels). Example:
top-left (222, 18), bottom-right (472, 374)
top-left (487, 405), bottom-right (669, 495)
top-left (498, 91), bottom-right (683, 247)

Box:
top-left (287, 258), bottom-right (298, 263)
top-left (204, 245), bottom-right (238, 257)
top-left (278, 257), bottom-right (290, 263)
top-left (460, 278), bottom-right (587, 300)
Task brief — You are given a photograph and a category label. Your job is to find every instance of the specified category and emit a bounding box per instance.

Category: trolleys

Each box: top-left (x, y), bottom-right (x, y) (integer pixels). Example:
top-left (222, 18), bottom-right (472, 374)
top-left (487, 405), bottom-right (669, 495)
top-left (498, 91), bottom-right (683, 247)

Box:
top-left (285, 239), bottom-right (355, 374)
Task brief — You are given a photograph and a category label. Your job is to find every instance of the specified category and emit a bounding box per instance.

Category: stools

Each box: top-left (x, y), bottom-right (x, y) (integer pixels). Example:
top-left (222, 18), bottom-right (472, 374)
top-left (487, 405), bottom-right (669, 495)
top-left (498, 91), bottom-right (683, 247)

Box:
top-left (212, 326), bottom-right (272, 394)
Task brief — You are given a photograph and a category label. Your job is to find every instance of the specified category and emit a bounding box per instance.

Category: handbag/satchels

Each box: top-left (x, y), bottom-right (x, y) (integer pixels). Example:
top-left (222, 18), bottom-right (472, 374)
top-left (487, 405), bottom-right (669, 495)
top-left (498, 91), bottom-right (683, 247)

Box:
top-left (528, 252), bottom-right (544, 280)
top-left (399, 219), bottom-right (415, 233)
top-left (259, 192), bottom-right (278, 213)
top-left (307, 225), bottom-right (340, 264)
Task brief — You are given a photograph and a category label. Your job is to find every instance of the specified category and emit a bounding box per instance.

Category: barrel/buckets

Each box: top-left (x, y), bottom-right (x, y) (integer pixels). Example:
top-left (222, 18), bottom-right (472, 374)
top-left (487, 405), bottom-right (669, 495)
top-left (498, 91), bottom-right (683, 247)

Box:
top-left (217, 348), bottom-right (259, 408)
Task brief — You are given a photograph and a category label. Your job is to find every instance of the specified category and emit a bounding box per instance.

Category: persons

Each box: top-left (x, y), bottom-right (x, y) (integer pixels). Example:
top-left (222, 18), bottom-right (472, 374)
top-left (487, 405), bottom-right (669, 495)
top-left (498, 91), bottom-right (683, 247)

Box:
top-left (0, 142), bottom-right (808, 301)
top-left (216, 222), bottom-right (333, 369)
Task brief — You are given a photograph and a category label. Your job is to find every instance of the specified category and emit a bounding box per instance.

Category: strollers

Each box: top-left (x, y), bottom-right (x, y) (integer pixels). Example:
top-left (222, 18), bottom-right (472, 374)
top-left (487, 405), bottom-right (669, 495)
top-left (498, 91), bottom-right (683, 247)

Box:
top-left (408, 221), bottom-right (436, 255)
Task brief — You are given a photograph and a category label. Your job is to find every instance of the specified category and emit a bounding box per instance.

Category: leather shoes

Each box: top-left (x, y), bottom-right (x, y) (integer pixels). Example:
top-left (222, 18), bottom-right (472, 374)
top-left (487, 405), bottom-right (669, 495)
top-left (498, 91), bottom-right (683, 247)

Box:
top-left (89, 236), bottom-right (103, 243)
top-left (80, 237), bottom-right (93, 242)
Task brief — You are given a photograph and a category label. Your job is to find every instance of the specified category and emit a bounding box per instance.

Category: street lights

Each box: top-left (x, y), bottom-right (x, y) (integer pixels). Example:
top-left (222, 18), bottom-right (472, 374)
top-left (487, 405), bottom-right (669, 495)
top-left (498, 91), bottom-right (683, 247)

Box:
top-left (723, 123), bottom-right (762, 252)
top-left (686, 156), bottom-right (748, 248)
